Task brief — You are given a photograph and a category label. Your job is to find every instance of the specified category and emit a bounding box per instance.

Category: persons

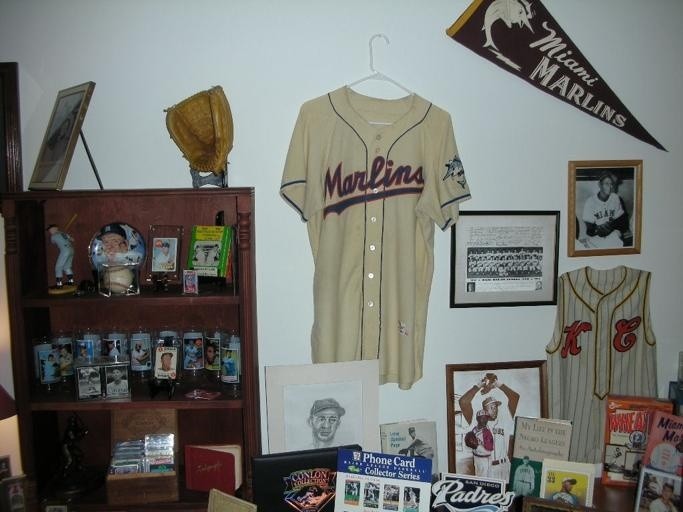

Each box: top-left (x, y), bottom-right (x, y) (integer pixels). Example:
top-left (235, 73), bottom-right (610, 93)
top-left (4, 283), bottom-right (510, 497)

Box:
top-left (646, 481), bottom-right (679, 511)
top-left (307, 397), bottom-right (345, 449)
top-left (512, 454), bottom-right (535, 495)
top-left (457, 372), bottom-right (521, 485)
top-left (45, 117), bottom-right (71, 161)
top-left (464, 409), bottom-right (494, 477)
top-left (61, 427), bottom-right (88, 477)
top-left (43, 223), bottom-right (76, 290)
top-left (344, 481), bottom-right (417, 509)
top-left (37, 336), bottom-right (237, 399)
top-left (91, 222), bottom-right (219, 294)
top-left (582, 170), bottom-right (629, 249)
top-left (467, 247), bottom-right (543, 292)
top-left (398, 425), bottom-right (433, 461)
top-left (548, 476), bottom-right (580, 507)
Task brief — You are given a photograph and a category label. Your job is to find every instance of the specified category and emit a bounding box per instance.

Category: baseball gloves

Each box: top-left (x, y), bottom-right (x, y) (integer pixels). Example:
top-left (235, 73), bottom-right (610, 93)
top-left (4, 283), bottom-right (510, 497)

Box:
top-left (480, 373), bottom-right (498, 395)
top-left (464, 432), bottom-right (478, 449)
top-left (163, 86), bottom-right (236, 189)
top-left (398, 448), bottom-right (408, 455)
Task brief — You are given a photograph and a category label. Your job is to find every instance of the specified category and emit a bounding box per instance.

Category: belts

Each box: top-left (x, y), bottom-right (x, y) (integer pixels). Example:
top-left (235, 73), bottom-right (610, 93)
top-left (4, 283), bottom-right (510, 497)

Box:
top-left (493, 458), bottom-right (508, 465)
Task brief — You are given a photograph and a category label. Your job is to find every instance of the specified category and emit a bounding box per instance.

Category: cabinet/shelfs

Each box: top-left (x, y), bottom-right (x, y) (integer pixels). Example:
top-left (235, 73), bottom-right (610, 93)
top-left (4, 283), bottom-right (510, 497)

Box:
top-left (13, 184), bottom-right (260, 512)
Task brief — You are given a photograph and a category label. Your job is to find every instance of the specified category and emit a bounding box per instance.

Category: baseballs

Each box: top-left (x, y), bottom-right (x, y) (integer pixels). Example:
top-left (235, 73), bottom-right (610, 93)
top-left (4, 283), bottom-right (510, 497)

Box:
top-left (485, 378), bottom-right (489, 386)
top-left (105, 266), bottom-right (133, 292)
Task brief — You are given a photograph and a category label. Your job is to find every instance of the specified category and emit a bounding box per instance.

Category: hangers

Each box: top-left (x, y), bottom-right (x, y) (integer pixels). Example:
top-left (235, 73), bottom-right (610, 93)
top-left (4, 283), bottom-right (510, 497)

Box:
top-left (345, 33), bottom-right (414, 125)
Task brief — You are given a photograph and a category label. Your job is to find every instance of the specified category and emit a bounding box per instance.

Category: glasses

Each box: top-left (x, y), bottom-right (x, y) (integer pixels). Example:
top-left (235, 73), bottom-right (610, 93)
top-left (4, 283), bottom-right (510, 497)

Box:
top-left (314, 416), bottom-right (340, 423)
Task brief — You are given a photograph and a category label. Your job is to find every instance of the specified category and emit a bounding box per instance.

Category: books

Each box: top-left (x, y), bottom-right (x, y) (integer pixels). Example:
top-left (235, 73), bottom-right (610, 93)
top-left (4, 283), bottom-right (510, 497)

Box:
top-left (599, 393), bottom-right (674, 485)
top-left (379, 418), bottom-right (439, 476)
top-left (181, 441), bottom-right (243, 496)
top-left (104, 471), bottom-right (181, 505)
top-left (223, 246), bottom-right (233, 284)
top-left (642, 410), bottom-right (683, 478)
top-left (110, 407), bottom-right (180, 473)
top-left (668, 381), bottom-right (682, 416)
top-left (333, 448), bottom-right (433, 512)
top-left (249, 443), bottom-right (362, 511)
top-left (0, 474), bottom-right (30, 512)
top-left (427, 470), bottom-right (516, 512)
top-left (507, 416), bottom-right (573, 498)
top-left (186, 224), bottom-right (233, 278)
top-left (206, 487), bottom-right (257, 511)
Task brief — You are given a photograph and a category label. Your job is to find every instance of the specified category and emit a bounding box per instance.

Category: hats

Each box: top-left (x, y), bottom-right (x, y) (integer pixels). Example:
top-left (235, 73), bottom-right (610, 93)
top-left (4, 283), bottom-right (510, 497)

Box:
top-left (96, 224), bottom-right (127, 240)
top-left (562, 478), bottom-right (577, 485)
top-left (311, 398), bottom-right (345, 417)
top-left (477, 410), bottom-right (490, 417)
top-left (482, 397), bottom-right (502, 406)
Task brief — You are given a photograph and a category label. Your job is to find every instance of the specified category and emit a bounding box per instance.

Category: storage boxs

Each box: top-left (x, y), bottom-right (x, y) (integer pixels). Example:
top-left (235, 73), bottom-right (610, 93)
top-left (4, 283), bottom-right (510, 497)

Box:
top-left (106, 406), bottom-right (178, 505)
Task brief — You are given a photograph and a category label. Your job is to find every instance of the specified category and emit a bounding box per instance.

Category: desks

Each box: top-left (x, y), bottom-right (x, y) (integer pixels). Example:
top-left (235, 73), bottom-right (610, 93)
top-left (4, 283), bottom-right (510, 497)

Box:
top-left (506, 477), bottom-right (640, 512)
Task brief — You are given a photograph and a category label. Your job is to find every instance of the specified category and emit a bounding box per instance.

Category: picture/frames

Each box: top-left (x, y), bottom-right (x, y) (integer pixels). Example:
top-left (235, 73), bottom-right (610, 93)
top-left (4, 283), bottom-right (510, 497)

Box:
top-left (446, 358), bottom-right (550, 473)
top-left (521, 497), bottom-right (586, 512)
top-left (567, 160), bottom-right (641, 256)
top-left (28, 80), bottom-right (104, 191)
top-left (449, 209), bottom-right (561, 307)
top-left (147, 224), bottom-right (183, 285)
